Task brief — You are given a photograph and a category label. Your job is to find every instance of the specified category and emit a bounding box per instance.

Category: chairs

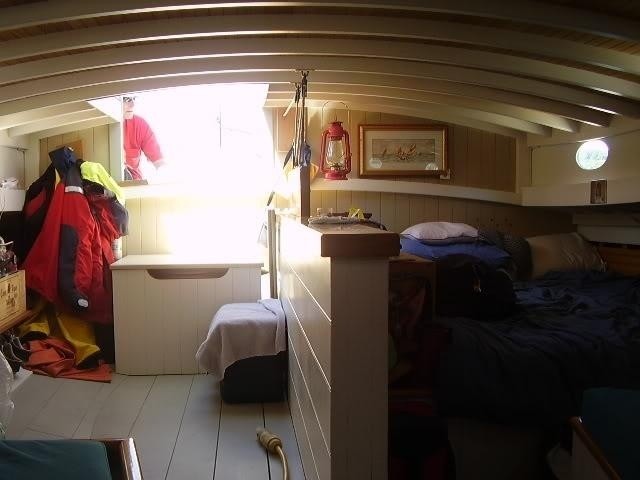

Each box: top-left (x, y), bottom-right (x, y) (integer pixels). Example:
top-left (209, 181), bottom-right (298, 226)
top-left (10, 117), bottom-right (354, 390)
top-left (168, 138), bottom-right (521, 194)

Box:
top-left (1, 349), bottom-right (144, 480)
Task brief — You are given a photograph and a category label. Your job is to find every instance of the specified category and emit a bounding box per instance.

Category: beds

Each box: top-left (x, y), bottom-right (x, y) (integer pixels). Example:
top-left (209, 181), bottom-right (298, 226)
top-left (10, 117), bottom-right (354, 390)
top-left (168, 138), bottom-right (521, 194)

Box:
top-left (389, 266), bottom-right (639, 476)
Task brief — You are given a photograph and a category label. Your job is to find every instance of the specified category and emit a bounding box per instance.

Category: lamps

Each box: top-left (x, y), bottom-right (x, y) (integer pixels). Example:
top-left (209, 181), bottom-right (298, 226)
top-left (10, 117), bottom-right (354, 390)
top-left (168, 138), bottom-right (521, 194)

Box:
top-left (319, 97), bottom-right (353, 182)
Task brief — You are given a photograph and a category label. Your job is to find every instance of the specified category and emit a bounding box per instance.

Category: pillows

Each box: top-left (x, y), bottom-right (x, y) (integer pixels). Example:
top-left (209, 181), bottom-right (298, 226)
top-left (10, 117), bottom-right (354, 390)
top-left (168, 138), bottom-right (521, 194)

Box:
top-left (398, 222), bottom-right (610, 280)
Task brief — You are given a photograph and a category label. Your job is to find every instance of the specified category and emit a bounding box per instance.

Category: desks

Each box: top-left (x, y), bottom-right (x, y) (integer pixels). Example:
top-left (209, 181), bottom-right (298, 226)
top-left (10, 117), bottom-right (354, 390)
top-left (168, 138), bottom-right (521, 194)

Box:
top-left (111, 252), bottom-right (265, 379)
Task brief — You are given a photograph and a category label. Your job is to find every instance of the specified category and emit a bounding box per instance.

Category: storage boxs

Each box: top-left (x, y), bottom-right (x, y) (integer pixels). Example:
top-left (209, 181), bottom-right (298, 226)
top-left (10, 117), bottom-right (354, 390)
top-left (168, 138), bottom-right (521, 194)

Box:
top-left (0, 268), bottom-right (28, 329)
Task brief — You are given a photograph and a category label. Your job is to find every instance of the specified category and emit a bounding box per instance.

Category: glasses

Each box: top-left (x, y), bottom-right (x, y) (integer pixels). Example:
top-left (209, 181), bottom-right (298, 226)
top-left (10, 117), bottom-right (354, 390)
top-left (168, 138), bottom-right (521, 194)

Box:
top-left (122, 96), bottom-right (137, 103)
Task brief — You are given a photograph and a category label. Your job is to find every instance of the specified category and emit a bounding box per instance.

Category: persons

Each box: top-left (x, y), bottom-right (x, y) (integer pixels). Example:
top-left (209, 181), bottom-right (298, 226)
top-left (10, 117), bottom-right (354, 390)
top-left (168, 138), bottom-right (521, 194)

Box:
top-left (123, 97), bottom-right (166, 181)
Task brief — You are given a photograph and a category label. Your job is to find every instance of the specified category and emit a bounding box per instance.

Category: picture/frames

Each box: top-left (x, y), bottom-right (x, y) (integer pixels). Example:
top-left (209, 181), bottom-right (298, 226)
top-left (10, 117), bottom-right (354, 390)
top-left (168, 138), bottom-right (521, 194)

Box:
top-left (357, 121), bottom-right (451, 179)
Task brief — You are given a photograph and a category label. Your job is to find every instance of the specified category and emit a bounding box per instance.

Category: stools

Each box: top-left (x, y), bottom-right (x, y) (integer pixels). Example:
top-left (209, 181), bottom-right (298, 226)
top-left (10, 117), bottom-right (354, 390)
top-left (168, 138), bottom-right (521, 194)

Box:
top-left (217, 301), bottom-right (288, 402)
